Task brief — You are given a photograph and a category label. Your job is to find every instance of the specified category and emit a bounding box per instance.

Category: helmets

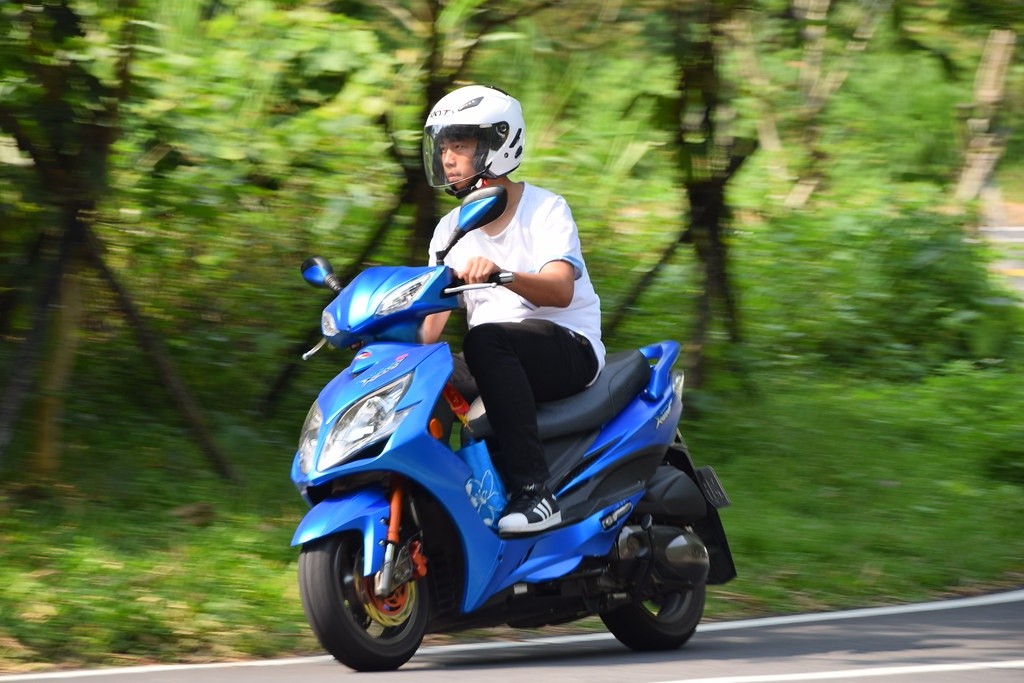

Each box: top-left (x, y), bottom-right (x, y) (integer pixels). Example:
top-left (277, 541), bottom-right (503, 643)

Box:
top-left (422, 85), bottom-right (525, 188)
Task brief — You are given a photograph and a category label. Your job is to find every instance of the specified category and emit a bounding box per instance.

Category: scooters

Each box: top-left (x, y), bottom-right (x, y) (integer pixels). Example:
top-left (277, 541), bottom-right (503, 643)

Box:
top-left (290, 184), bottom-right (738, 672)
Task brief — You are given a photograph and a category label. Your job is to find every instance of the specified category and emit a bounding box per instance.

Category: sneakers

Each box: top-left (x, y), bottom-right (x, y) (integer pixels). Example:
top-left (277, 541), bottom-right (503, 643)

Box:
top-left (497, 486), bottom-right (562, 538)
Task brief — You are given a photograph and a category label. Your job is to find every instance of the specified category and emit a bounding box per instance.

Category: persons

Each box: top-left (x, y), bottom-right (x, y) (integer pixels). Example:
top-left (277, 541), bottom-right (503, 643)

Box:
top-left (421, 84), bottom-right (605, 533)
